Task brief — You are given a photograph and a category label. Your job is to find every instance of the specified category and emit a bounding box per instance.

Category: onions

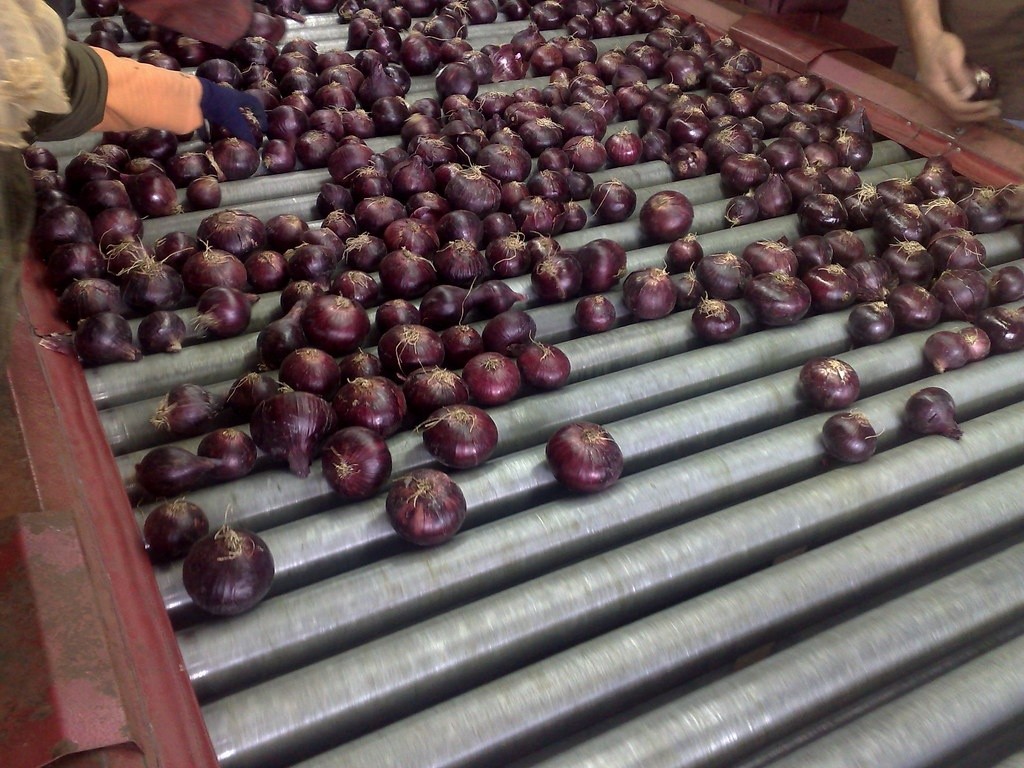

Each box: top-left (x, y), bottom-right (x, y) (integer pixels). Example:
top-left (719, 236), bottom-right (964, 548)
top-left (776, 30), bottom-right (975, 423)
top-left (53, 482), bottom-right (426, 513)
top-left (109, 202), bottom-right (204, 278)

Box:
top-left (18, 0), bottom-right (1024, 618)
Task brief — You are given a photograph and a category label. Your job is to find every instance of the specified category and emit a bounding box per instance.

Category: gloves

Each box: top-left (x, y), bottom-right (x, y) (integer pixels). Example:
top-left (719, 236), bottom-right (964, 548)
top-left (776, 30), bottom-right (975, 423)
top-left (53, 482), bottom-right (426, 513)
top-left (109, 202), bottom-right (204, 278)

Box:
top-left (198, 77), bottom-right (268, 144)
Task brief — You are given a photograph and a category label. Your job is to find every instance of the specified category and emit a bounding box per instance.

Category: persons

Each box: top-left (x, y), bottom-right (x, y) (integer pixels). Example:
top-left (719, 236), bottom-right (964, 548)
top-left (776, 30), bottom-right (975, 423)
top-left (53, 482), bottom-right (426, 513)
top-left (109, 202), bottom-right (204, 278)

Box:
top-left (902, 0), bottom-right (1024, 122)
top-left (0, 0), bottom-right (267, 365)
